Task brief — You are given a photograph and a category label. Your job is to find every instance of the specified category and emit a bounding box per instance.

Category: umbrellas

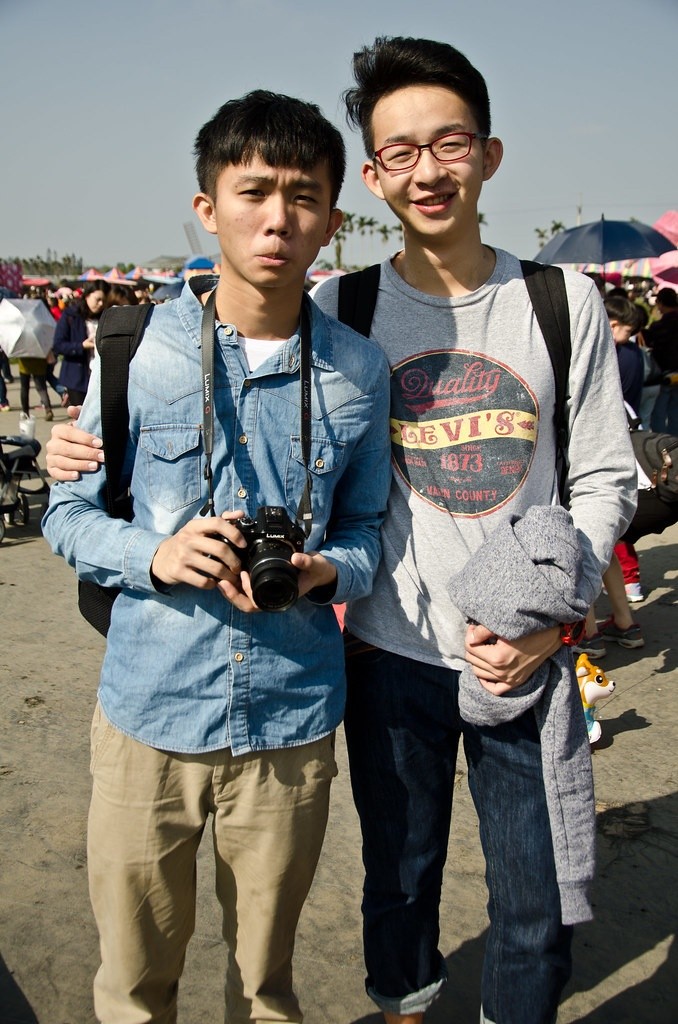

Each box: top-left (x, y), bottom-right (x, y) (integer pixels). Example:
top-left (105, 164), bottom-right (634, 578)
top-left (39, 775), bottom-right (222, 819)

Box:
top-left (0, 297), bottom-right (57, 360)
top-left (531, 214), bottom-right (678, 274)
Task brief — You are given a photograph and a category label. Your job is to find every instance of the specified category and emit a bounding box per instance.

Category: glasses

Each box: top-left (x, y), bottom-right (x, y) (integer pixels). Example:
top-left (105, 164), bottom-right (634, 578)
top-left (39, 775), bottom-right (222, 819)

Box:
top-left (373, 131), bottom-right (487, 171)
top-left (19, 411), bottom-right (37, 441)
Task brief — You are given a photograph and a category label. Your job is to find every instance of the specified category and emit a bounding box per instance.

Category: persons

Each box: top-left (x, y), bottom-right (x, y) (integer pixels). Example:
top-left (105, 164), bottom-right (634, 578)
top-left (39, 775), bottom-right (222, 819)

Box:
top-left (0, 279), bottom-right (154, 420)
top-left (570, 280), bottom-right (678, 660)
top-left (46, 37), bottom-right (639, 1024)
top-left (40, 88), bottom-right (392, 1024)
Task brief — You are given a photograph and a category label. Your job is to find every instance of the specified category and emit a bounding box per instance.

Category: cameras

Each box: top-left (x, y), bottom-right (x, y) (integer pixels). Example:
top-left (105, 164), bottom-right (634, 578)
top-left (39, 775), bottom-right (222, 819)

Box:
top-left (210, 506), bottom-right (304, 613)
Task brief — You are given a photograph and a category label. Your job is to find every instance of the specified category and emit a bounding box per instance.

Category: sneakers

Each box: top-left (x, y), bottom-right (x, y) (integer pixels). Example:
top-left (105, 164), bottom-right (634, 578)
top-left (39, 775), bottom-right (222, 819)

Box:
top-left (596, 613), bottom-right (644, 649)
top-left (602, 582), bottom-right (644, 602)
top-left (571, 630), bottom-right (606, 659)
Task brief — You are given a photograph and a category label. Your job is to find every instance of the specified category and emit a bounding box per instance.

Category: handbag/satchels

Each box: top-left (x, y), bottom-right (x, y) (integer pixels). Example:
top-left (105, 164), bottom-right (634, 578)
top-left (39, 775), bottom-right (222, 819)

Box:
top-left (77, 300), bottom-right (152, 639)
top-left (624, 415), bottom-right (677, 504)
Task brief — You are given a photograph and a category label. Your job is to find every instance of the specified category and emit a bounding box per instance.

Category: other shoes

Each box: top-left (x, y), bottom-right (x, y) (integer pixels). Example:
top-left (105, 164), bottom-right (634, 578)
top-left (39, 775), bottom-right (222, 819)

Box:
top-left (45, 411), bottom-right (54, 421)
top-left (61, 392), bottom-right (70, 407)
top-left (0, 403), bottom-right (11, 411)
